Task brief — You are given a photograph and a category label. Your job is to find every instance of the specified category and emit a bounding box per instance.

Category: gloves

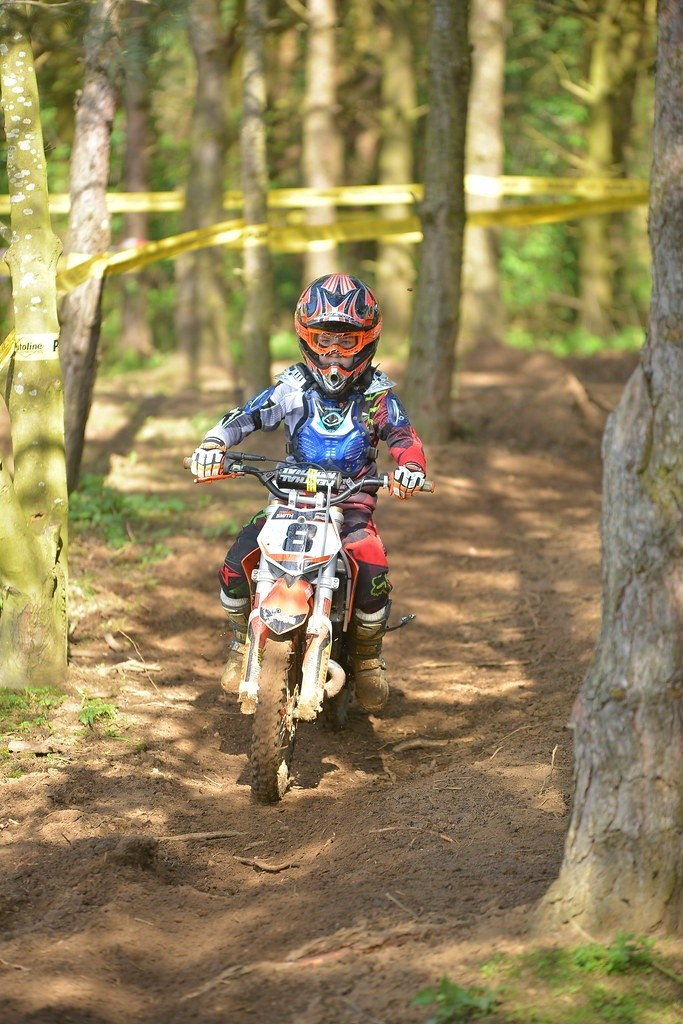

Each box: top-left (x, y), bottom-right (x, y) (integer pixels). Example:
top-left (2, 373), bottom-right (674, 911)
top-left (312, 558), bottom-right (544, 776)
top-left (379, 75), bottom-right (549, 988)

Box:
top-left (393, 463), bottom-right (426, 499)
top-left (191, 438), bottom-right (224, 483)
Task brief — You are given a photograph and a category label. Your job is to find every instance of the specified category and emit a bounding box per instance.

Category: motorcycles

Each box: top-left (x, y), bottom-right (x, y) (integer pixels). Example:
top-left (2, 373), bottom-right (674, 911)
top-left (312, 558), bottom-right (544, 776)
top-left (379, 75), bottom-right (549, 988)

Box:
top-left (183, 450), bottom-right (434, 803)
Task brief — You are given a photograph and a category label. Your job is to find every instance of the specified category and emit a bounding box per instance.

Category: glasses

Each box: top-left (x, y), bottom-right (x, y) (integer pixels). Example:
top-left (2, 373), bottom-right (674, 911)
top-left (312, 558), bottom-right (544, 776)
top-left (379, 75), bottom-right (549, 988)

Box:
top-left (293, 315), bottom-right (381, 358)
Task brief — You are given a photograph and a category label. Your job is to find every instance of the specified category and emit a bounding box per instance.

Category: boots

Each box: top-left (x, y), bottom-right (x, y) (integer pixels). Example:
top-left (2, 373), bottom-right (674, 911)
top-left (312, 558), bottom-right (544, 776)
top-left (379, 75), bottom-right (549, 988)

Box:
top-left (350, 598), bottom-right (389, 713)
top-left (221, 601), bottom-right (248, 694)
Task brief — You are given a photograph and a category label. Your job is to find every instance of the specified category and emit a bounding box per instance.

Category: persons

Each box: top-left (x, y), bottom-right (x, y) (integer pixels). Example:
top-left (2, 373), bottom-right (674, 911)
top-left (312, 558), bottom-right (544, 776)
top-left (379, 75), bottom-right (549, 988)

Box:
top-left (192, 274), bottom-right (427, 713)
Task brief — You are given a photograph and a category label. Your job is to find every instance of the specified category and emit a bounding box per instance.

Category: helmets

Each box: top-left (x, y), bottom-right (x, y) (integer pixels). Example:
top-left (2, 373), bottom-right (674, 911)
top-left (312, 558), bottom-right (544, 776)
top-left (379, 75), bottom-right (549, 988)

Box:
top-left (293, 272), bottom-right (382, 397)
